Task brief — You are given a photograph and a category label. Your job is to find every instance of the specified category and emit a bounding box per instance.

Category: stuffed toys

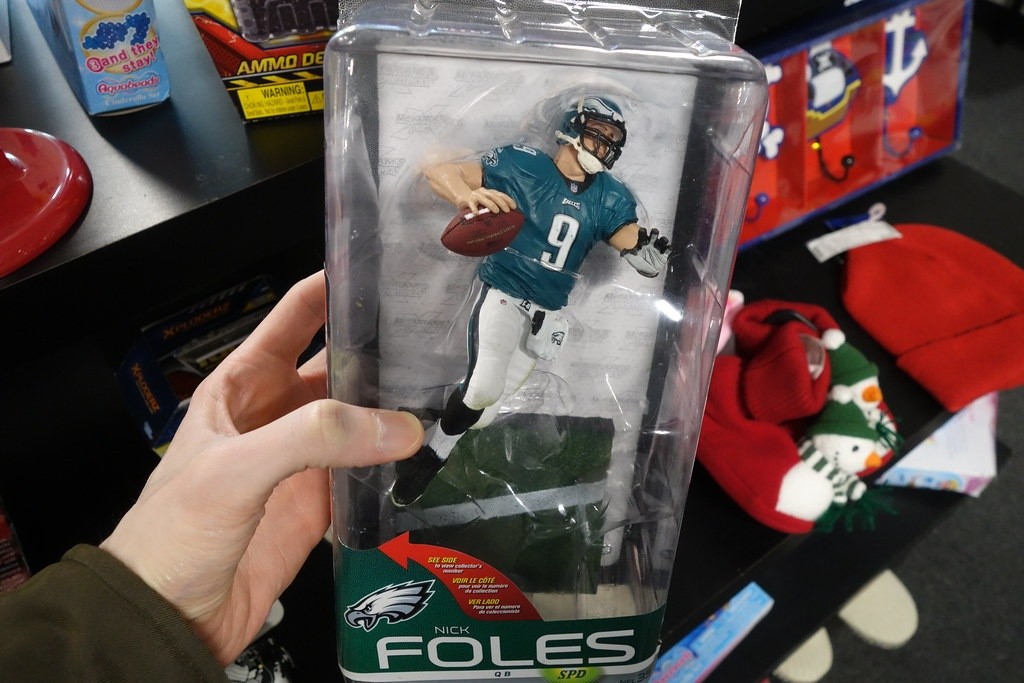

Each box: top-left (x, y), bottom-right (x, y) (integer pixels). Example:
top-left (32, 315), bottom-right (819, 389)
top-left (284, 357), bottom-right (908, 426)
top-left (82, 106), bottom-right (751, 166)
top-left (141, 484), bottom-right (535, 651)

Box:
top-left (695, 300), bottom-right (900, 534)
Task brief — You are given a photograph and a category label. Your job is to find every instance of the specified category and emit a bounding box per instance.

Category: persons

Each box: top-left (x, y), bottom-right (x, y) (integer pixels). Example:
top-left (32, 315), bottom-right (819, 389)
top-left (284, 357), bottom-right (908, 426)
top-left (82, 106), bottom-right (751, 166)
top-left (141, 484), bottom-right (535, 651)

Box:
top-left (0, 262), bottom-right (423, 683)
top-left (391, 97), bottom-right (674, 505)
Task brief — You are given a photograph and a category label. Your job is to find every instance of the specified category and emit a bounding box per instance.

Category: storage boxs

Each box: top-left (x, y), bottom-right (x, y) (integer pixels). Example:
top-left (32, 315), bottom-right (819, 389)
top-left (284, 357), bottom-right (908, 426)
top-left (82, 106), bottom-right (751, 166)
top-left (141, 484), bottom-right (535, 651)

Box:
top-left (40, 0), bottom-right (169, 116)
top-left (182, 0), bottom-right (338, 126)
top-left (309, 0), bottom-right (767, 683)
top-left (739, 0), bottom-right (969, 252)
